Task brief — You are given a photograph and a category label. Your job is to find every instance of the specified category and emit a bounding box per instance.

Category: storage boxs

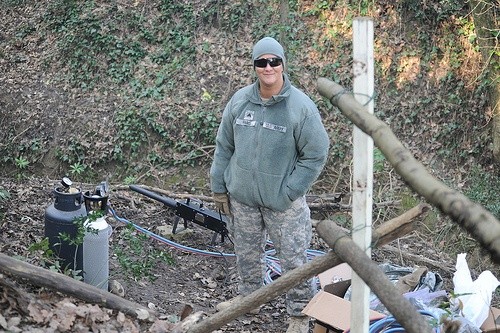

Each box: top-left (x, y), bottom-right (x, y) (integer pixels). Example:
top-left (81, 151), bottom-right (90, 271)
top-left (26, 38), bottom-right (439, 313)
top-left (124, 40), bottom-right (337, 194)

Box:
top-left (301, 262), bottom-right (387, 333)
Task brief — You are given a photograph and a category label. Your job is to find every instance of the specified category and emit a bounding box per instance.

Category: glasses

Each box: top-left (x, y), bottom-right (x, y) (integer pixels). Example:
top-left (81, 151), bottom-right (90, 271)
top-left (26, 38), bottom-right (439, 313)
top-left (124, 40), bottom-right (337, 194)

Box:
top-left (254, 58), bottom-right (282, 68)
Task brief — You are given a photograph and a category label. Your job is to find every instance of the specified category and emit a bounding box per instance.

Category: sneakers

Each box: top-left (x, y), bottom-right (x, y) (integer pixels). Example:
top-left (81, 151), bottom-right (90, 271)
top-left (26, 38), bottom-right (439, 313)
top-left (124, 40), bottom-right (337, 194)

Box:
top-left (286, 317), bottom-right (309, 333)
top-left (216, 295), bottom-right (261, 315)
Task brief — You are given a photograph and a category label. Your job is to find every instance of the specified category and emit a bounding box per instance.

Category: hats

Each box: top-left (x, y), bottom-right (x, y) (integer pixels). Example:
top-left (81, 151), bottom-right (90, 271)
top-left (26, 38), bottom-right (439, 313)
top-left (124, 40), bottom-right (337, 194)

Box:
top-left (252, 37), bottom-right (286, 71)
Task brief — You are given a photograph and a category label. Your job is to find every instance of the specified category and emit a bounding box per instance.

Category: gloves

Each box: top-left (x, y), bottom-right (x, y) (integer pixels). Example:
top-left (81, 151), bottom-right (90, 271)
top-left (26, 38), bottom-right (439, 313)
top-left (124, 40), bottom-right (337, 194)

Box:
top-left (212, 193), bottom-right (230, 216)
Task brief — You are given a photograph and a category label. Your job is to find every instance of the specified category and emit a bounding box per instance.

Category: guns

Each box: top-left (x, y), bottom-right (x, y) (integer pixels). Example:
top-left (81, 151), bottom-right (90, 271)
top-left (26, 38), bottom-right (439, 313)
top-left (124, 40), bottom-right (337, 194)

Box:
top-left (128, 184), bottom-right (234, 245)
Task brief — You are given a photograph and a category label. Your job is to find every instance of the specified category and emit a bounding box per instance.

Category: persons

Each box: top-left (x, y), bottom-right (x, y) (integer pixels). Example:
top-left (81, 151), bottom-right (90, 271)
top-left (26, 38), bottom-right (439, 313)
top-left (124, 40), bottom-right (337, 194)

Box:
top-left (209, 37), bottom-right (330, 333)
